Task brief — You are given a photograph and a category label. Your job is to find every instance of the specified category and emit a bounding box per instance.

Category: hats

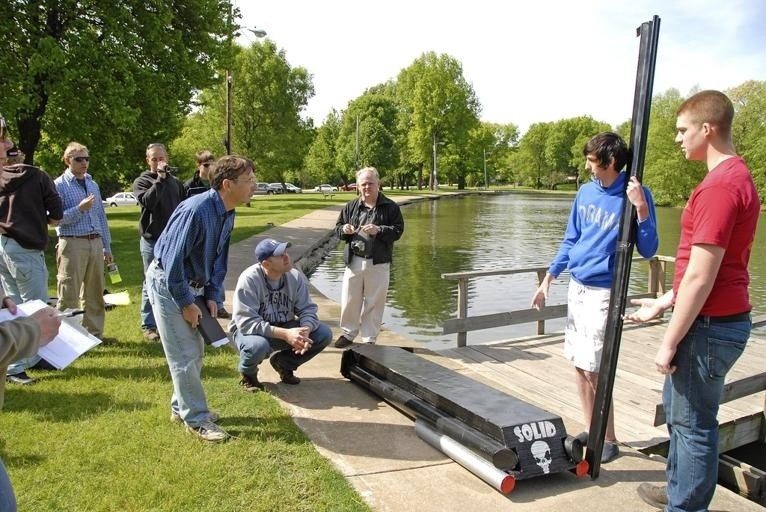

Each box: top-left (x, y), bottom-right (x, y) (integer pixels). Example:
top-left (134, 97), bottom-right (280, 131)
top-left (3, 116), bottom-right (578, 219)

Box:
top-left (7, 142), bottom-right (18, 156)
top-left (253, 238), bottom-right (291, 263)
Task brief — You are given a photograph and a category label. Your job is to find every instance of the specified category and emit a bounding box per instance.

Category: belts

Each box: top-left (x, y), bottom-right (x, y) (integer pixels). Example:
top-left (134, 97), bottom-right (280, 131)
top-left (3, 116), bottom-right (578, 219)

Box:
top-left (351, 252), bottom-right (373, 259)
top-left (60, 234), bottom-right (101, 240)
top-left (184, 278), bottom-right (206, 290)
top-left (672, 305), bottom-right (751, 323)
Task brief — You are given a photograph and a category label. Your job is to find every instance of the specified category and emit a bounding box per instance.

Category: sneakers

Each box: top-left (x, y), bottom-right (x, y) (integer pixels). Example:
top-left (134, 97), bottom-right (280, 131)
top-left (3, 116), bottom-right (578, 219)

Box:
top-left (99, 337), bottom-right (116, 346)
top-left (170, 411), bottom-right (220, 424)
top-left (269, 351), bottom-right (300, 384)
top-left (637, 481), bottom-right (669, 509)
top-left (217, 308), bottom-right (229, 318)
top-left (573, 431), bottom-right (589, 446)
top-left (6, 372), bottom-right (37, 386)
top-left (335, 335), bottom-right (353, 348)
top-left (600, 442), bottom-right (619, 463)
top-left (27, 358), bottom-right (58, 371)
top-left (185, 421), bottom-right (229, 444)
top-left (142, 328), bottom-right (159, 341)
top-left (240, 376), bottom-right (263, 392)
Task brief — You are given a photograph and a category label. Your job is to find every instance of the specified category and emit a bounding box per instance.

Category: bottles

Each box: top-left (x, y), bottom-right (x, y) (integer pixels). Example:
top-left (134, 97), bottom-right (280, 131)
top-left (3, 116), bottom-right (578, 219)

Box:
top-left (106, 259), bottom-right (122, 284)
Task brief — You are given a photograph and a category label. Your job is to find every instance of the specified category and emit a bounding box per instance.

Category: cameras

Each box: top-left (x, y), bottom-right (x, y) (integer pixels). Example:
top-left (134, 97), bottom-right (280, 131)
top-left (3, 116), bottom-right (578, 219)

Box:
top-left (164, 165), bottom-right (179, 173)
top-left (350, 240), bottom-right (367, 254)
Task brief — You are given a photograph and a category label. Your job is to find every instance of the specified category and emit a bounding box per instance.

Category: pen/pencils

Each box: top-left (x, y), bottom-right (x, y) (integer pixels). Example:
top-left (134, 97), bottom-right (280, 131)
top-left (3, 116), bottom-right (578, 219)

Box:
top-left (50, 310), bottom-right (87, 318)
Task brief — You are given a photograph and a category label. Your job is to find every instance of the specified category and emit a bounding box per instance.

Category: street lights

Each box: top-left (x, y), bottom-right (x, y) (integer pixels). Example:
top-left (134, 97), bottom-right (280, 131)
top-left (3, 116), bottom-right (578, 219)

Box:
top-left (483, 147), bottom-right (493, 188)
top-left (433, 141), bottom-right (446, 191)
top-left (224, 25), bottom-right (268, 157)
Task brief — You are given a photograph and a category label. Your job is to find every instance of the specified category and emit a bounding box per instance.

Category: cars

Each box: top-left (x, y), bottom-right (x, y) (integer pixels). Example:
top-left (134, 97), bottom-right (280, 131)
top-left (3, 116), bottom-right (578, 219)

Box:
top-left (105, 192), bottom-right (139, 207)
top-left (315, 184), bottom-right (338, 192)
top-left (253, 183), bottom-right (302, 194)
top-left (338, 182), bottom-right (357, 191)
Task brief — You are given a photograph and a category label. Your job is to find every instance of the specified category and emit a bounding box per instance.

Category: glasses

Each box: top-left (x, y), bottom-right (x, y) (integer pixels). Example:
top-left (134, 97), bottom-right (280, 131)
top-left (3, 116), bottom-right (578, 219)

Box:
top-left (229, 177), bottom-right (257, 184)
top-left (68, 156), bottom-right (90, 162)
top-left (200, 164), bottom-right (210, 168)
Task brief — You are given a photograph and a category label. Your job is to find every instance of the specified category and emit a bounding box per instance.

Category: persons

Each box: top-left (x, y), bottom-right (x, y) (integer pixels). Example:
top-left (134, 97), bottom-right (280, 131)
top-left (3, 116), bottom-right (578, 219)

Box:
top-left (620, 90), bottom-right (760, 511)
top-left (183, 148), bottom-right (232, 318)
top-left (531, 132), bottom-right (656, 462)
top-left (1, 145), bottom-right (64, 384)
top-left (230, 238), bottom-right (331, 391)
top-left (51, 142), bottom-right (117, 346)
top-left (333, 166), bottom-right (405, 348)
top-left (1, 111), bottom-right (59, 510)
top-left (133, 143), bottom-right (187, 340)
top-left (145, 156), bottom-right (257, 442)
